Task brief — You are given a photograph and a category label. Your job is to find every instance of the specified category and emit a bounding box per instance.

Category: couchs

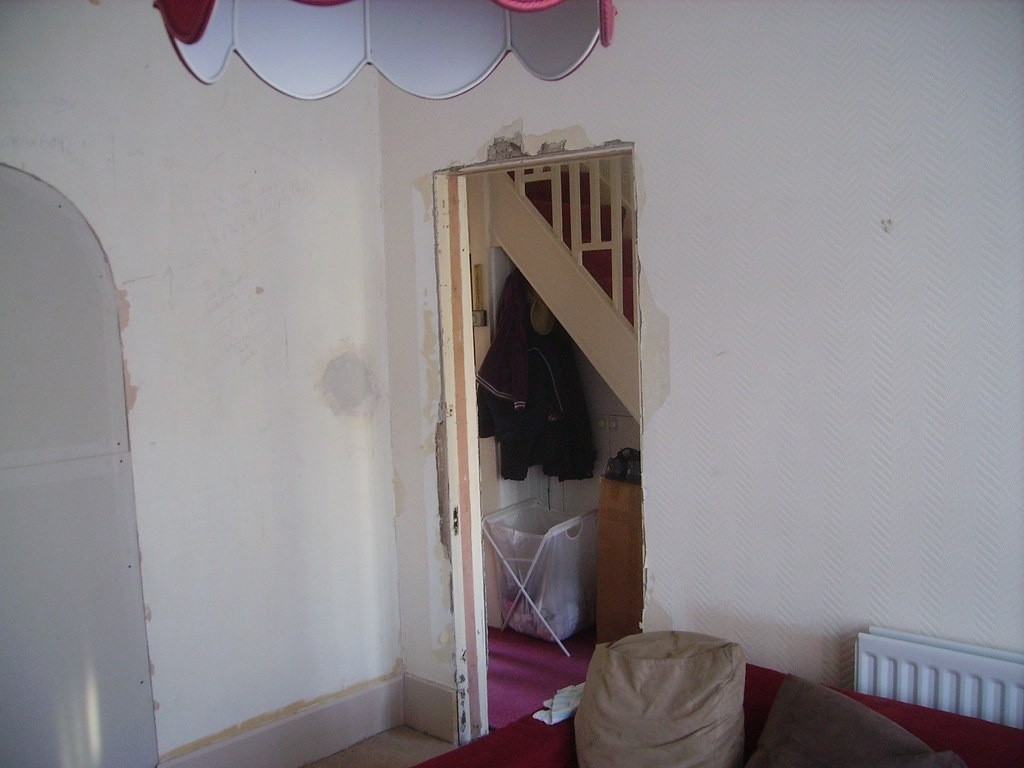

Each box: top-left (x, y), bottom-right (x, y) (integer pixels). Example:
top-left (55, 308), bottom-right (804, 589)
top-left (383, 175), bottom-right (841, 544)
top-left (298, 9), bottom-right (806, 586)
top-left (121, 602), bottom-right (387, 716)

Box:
top-left (411, 663), bottom-right (1024, 768)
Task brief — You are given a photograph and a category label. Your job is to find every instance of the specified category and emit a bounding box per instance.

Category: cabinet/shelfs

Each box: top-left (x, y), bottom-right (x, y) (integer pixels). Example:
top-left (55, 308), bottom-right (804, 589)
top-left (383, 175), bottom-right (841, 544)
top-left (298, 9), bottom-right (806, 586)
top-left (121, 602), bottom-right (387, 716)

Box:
top-left (563, 413), bottom-right (640, 515)
top-left (596, 475), bottom-right (644, 643)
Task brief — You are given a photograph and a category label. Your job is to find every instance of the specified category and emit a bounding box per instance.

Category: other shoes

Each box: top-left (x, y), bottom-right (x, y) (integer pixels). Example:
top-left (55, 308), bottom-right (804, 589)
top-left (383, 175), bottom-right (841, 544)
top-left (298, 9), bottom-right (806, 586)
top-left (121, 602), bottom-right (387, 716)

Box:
top-left (607, 448), bottom-right (635, 479)
top-left (627, 452), bottom-right (641, 484)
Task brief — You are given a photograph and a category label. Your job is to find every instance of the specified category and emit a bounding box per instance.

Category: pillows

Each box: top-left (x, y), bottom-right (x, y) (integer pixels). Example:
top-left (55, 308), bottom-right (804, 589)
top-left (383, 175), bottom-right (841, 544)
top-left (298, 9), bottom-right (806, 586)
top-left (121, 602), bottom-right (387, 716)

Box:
top-left (747, 673), bottom-right (968, 768)
top-left (574, 632), bottom-right (746, 768)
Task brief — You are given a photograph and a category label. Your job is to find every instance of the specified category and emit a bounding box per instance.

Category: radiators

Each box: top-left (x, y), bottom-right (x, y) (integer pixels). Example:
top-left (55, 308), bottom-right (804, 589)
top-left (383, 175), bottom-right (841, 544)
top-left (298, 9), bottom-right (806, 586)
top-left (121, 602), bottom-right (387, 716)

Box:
top-left (853, 626), bottom-right (1023, 730)
